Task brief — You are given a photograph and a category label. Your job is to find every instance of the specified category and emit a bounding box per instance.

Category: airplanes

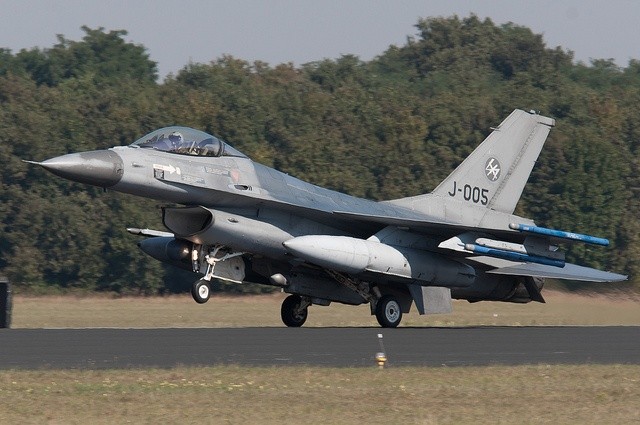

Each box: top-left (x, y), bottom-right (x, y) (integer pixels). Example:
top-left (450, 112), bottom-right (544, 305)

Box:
top-left (20, 108), bottom-right (628, 328)
